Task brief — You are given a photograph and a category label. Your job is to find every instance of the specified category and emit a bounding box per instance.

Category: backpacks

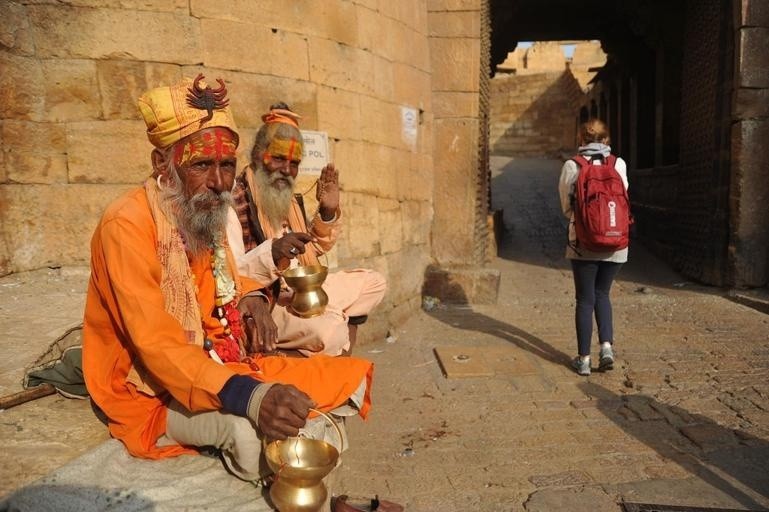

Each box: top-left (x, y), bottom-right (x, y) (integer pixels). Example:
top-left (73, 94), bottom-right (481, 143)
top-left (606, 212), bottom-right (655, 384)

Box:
top-left (569, 154), bottom-right (635, 252)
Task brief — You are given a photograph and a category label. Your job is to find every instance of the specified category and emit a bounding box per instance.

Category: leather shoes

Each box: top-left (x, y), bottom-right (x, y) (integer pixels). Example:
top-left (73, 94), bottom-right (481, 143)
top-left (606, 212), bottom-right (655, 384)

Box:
top-left (334, 495), bottom-right (404, 512)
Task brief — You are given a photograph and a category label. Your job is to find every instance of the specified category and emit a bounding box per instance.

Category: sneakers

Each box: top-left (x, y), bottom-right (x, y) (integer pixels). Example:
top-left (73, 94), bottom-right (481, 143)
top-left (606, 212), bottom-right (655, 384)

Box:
top-left (572, 357), bottom-right (591, 375)
top-left (599, 348), bottom-right (614, 369)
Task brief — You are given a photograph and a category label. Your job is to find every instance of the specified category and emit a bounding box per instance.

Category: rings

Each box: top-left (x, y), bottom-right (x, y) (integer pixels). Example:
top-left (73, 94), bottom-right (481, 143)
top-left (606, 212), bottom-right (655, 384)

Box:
top-left (244, 314), bottom-right (255, 323)
top-left (292, 247), bottom-right (297, 254)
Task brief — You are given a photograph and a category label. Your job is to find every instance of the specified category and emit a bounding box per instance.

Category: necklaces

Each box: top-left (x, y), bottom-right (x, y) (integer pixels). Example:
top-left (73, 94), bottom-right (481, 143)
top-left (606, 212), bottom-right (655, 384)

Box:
top-left (296, 179), bottom-right (326, 232)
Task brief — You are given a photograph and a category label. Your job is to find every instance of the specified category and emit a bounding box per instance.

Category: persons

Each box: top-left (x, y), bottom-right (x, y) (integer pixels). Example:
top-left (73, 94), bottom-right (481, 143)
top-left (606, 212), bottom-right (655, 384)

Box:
top-left (80, 74), bottom-right (373, 487)
top-left (558, 118), bottom-right (634, 377)
top-left (234, 103), bottom-right (387, 358)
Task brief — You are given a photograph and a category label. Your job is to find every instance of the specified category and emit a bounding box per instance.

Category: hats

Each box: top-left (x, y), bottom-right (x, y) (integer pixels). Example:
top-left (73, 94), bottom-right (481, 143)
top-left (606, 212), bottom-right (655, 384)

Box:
top-left (138, 72), bottom-right (239, 152)
